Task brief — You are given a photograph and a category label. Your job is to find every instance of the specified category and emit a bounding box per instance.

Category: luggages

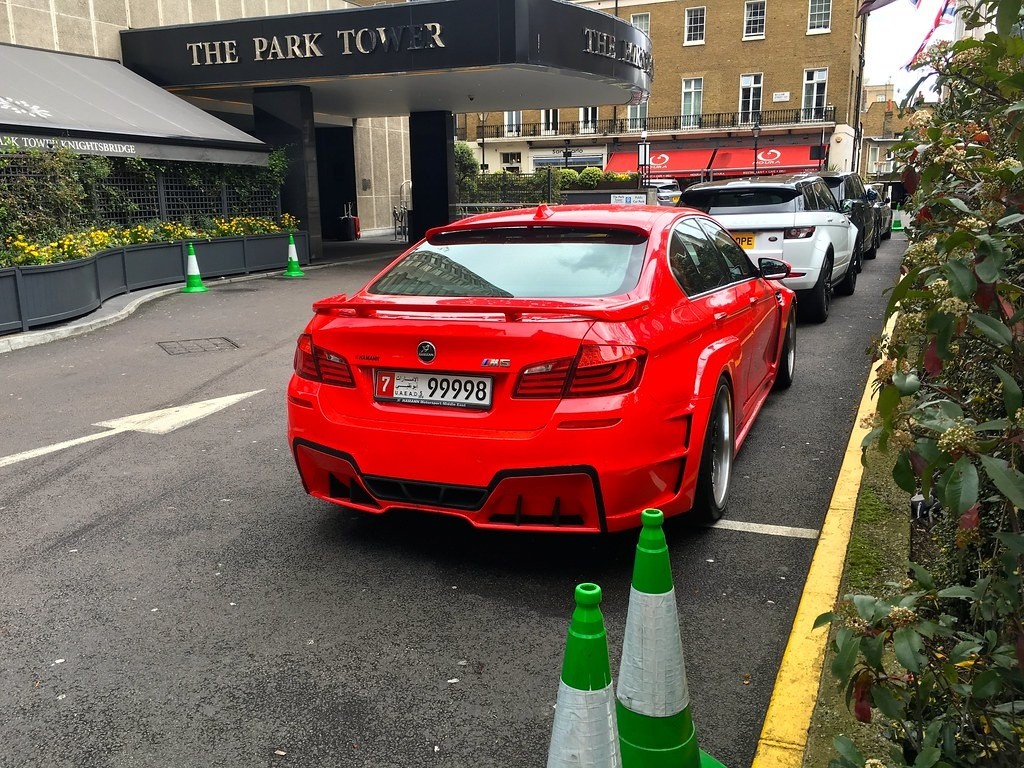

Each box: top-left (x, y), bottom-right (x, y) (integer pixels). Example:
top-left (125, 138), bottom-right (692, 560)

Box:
top-left (339, 201), bottom-right (360, 241)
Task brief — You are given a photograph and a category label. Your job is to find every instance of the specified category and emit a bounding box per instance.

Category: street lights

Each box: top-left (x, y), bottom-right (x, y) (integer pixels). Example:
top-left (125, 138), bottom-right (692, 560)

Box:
top-left (476, 111), bottom-right (488, 199)
top-left (749, 122), bottom-right (762, 176)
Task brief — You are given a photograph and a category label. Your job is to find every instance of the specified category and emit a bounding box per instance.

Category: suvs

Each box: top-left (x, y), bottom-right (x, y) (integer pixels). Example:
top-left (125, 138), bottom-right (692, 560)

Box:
top-left (767, 172), bottom-right (880, 274)
top-left (667, 173), bottom-right (859, 327)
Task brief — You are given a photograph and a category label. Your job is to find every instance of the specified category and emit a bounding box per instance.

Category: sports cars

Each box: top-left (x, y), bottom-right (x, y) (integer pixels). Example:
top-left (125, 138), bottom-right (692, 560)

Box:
top-left (284, 200), bottom-right (795, 537)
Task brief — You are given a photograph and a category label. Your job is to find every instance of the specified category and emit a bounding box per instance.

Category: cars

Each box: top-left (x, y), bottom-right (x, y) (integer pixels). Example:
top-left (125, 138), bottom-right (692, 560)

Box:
top-left (863, 184), bottom-right (893, 247)
top-left (642, 179), bottom-right (682, 206)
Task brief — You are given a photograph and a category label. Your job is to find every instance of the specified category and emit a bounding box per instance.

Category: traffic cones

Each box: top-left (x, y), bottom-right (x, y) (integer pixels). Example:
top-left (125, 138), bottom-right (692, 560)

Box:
top-left (280, 233), bottom-right (305, 277)
top-left (178, 241), bottom-right (208, 293)
top-left (616, 507), bottom-right (730, 768)
top-left (543, 582), bottom-right (623, 768)
